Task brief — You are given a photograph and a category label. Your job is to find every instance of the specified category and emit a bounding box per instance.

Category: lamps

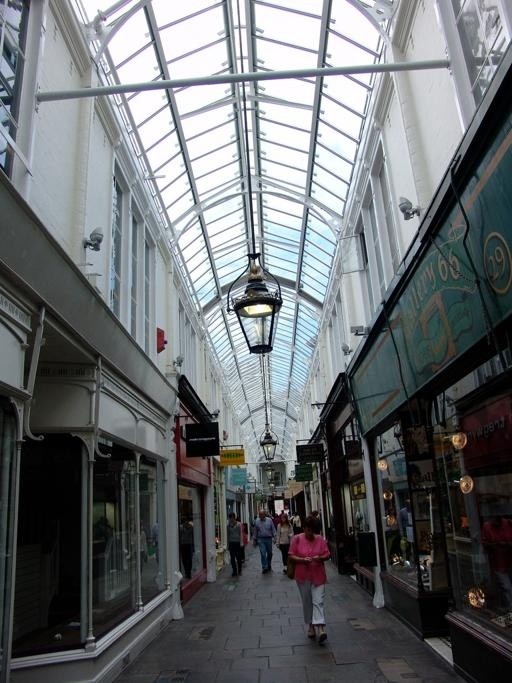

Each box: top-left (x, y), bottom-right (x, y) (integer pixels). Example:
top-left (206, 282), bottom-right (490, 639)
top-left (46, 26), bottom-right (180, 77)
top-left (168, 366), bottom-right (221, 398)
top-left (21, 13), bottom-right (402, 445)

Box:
top-left (172, 355), bottom-right (185, 368)
top-left (399, 196), bottom-right (422, 221)
top-left (82, 224), bottom-right (104, 252)
top-left (259, 357), bottom-right (279, 485)
top-left (341, 326), bottom-right (366, 357)
top-left (227, 2), bottom-right (284, 357)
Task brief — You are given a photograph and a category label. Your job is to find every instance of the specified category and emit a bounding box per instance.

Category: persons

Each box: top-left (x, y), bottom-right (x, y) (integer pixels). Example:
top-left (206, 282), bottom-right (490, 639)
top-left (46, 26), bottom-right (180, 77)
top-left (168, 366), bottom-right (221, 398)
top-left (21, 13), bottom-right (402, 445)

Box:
top-left (356, 508), bottom-right (363, 530)
top-left (252, 509), bottom-right (276, 574)
top-left (288, 516), bottom-right (332, 643)
top-left (481, 505), bottom-right (512, 574)
top-left (385, 498), bottom-right (416, 564)
top-left (94, 514), bottom-right (193, 580)
top-left (236, 518), bottom-right (248, 563)
top-left (276, 514), bottom-right (294, 573)
top-left (249, 503), bottom-right (324, 546)
top-left (226, 511), bottom-right (244, 577)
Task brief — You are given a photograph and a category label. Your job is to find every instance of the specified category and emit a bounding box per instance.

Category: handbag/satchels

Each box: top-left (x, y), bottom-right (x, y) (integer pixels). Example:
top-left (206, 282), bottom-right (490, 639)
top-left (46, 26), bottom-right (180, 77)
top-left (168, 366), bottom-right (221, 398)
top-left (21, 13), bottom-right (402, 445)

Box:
top-left (287, 558), bottom-right (295, 579)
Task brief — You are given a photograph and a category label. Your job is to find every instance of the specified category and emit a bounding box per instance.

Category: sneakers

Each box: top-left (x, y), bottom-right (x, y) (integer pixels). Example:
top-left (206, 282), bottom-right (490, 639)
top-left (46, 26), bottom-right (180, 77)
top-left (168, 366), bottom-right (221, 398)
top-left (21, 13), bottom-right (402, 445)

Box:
top-left (308, 627), bottom-right (316, 638)
top-left (317, 630), bottom-right (327, 644)
